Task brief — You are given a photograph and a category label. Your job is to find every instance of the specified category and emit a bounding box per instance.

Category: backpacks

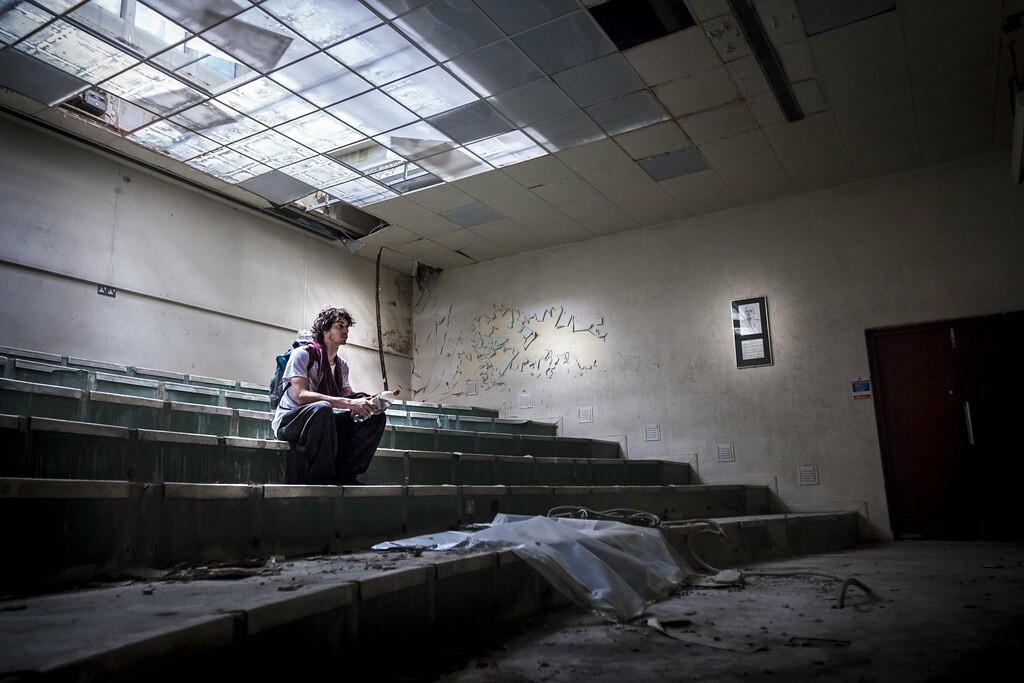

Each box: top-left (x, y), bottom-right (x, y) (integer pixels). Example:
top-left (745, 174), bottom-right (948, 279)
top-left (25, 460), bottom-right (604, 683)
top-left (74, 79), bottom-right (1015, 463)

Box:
top-left (269, 341), bottom-right (315, 410)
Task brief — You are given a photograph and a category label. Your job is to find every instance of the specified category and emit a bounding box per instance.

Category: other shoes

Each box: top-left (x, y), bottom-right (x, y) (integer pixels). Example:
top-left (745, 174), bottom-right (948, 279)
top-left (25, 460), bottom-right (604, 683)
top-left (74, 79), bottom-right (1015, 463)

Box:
top-left (307, 478), bottom-right (333, 485)
top-left (335, 474), bottom-right (365, 485)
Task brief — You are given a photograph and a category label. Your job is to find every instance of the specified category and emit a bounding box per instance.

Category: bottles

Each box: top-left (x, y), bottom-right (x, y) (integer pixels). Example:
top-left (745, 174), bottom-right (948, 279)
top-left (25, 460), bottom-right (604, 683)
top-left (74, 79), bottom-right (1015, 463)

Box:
top-left (351, 389), bottom-right (401, 423)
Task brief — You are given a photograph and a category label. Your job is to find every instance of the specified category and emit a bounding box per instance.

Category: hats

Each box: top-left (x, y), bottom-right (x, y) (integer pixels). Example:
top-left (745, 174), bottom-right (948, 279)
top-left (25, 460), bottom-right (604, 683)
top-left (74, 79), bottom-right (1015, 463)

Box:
top-left (295, 329), bottom-right (314, 342)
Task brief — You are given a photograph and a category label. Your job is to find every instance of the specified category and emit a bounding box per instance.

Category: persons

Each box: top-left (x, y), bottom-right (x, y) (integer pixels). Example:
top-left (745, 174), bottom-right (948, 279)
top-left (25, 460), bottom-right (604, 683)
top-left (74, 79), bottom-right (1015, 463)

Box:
top-left (271, 306), bottom-right (391, 485)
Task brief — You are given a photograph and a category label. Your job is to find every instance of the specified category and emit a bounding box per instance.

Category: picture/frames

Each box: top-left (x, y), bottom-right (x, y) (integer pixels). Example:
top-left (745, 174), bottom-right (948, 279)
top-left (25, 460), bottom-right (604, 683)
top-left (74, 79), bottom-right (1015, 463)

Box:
top-left (731, 296), bottom-right (773, 369)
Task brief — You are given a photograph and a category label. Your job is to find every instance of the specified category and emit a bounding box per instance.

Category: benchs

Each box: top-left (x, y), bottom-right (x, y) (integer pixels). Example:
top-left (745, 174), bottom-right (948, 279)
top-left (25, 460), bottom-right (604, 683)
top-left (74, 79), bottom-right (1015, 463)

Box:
top-left (0, 345), bottom-right (857, 683)
